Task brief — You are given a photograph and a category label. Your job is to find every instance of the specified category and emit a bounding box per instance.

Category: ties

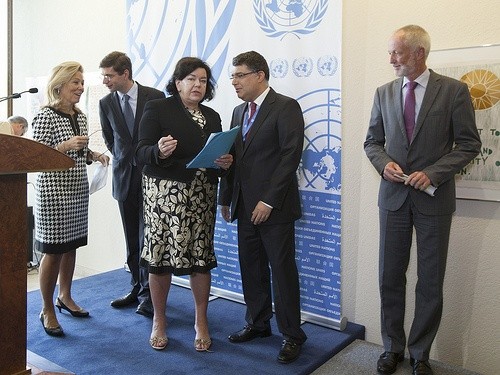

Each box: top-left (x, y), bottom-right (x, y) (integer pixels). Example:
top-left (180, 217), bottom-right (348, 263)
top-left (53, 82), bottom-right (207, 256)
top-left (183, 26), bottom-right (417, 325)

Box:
top-left (122, 94), bottom-right (137, 166)
top-left (404, 81), bottom-right (419, 145)
top-left (244, 102), bottom-right (257, 140)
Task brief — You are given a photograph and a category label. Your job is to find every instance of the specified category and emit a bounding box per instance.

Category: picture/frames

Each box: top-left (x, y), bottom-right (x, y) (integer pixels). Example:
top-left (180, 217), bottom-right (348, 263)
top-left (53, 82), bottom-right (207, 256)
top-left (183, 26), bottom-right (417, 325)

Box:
top-left (425, 43), bottom-right (500, 202)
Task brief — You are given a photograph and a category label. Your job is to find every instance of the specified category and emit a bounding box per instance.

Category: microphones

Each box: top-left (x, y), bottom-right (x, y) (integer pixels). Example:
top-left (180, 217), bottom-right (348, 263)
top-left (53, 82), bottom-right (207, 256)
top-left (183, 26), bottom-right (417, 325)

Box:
top-left (0, 88), bottom-right (39, 102)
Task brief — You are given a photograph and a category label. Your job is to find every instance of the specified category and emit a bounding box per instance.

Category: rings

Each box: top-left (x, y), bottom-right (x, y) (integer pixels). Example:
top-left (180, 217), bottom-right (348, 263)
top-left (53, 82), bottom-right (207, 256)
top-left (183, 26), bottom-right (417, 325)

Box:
top-left (161, 144), bottom-right (166, 148)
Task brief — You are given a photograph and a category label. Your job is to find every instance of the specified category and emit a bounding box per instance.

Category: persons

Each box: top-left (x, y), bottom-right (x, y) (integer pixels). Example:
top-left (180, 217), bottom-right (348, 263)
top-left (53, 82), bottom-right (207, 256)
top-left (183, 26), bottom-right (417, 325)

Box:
top-left (219, 51), bottom-right (307, 362)
top-left (363, 25), bottom-right (482, 375)
top-left (0, 116), bottom-right (29, 136)
top-left (135, 56), bottom-right (233, 350)
top-left (32, 61), bottom-right (109, 336)
top-left (99, 51), bottom-right (166, 315)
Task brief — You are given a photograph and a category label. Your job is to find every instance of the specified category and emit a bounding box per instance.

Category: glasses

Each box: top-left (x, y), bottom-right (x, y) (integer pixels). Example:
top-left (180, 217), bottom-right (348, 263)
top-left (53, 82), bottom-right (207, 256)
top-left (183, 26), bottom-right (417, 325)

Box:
top-left (229, 70), bottom-right (260, 81)
top-left (99, 72), bottom-right (119, 81)
top-left (84, 130), bottom-right (113, 162)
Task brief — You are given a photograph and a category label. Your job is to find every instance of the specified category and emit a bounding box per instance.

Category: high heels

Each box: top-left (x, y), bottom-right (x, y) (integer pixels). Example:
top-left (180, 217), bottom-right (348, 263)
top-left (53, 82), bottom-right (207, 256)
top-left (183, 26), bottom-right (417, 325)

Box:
top-left (55, 296), bottom-right (89, 318)
top-left (39, 311), bottom-right (63, 337)
top-left (194, 324), bottom-right (211, 352)
top-left (149, 337), bottom-right (168, 349)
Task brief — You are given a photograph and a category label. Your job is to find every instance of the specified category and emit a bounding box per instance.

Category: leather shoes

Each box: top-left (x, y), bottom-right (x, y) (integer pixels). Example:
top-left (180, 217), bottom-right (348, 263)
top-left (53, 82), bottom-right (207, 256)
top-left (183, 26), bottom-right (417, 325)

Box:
top-left (111, 292), bottom-right (138, 308)
top-left (136, 301), bottom-right (154, 316)
top-left (410, 356), bottom-right (432, 375)
top-left (377, 351), bottom-right (405, 375)
top-left (277, 340), bottom-right (302, 364)
top-left (228, 323), bottom-right (272, 342)
